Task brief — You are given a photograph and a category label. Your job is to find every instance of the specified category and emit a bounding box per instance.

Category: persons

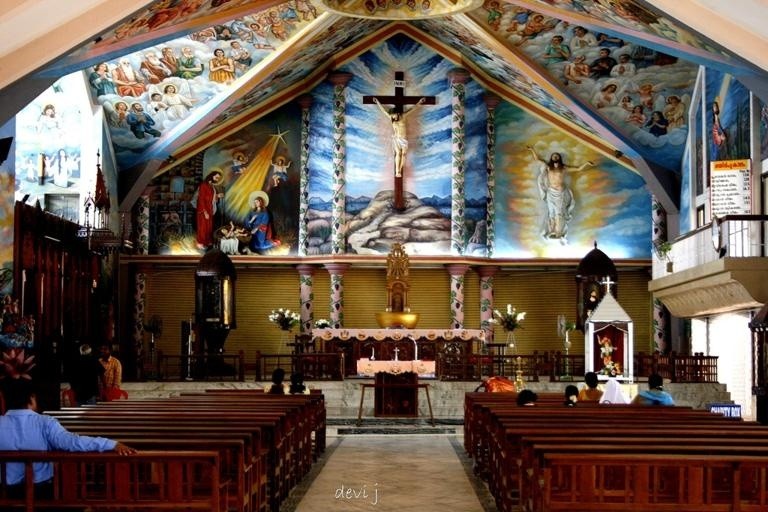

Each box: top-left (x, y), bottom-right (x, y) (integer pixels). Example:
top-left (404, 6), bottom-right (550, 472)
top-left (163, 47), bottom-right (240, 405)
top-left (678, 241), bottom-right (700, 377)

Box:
top-left (0, 378), bottom-right (138, 501)
top-left (263, 368), bottom-right (288, 393)
top-left (563, 385), bottom-right (579, 406)
top-left (373, 97), bottom-right (426, 177)
top-left (195, 152), bottom-right (292, 251)
top-left (15, 149), bottom-right (80, 188)
top-left (600, 343), bottom-right (619, 375)
top-left (364, 0), bottom-right (430, 12)
top-left (283, 371), bottom-right (311, 394)
top-left (598, 379), bottom-right (631, 404)
top-left (578, 371), bottom-right (604, 401)
top-left (97, 341), bottom-right (122, 389)
top-left (89, 0), bottom-right (317, 140)
top-left (482, 0), bottom-right (685, 138)
top-left (70, 343), bottom-right (106, 407)
top-left (526, 145), bottom-right (595, 238)
top-left (36, 100), bottom-right (59, 134)
top-left (516, 390), bottom-right (538, 405)
top-left (630, 372), bottom-right (675, 406)
top-left (584, 291), bottom-right (598, 315)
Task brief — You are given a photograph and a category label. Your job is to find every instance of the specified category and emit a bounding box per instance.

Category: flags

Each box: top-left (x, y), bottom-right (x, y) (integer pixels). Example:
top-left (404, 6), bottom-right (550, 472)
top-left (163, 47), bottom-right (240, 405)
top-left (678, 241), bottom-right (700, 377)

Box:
top-left (713, 122), bottom-right (726, 146)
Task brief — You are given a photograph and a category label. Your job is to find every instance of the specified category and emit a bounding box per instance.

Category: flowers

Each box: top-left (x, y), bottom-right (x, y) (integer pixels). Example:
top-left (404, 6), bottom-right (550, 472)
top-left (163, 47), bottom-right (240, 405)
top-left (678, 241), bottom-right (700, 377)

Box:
top-left (315, 319), bottom-right (332, 329)
top-left (268, 309), bottom-right (301, 331)
top-left (489, 304), bottom-right (527, 332)
